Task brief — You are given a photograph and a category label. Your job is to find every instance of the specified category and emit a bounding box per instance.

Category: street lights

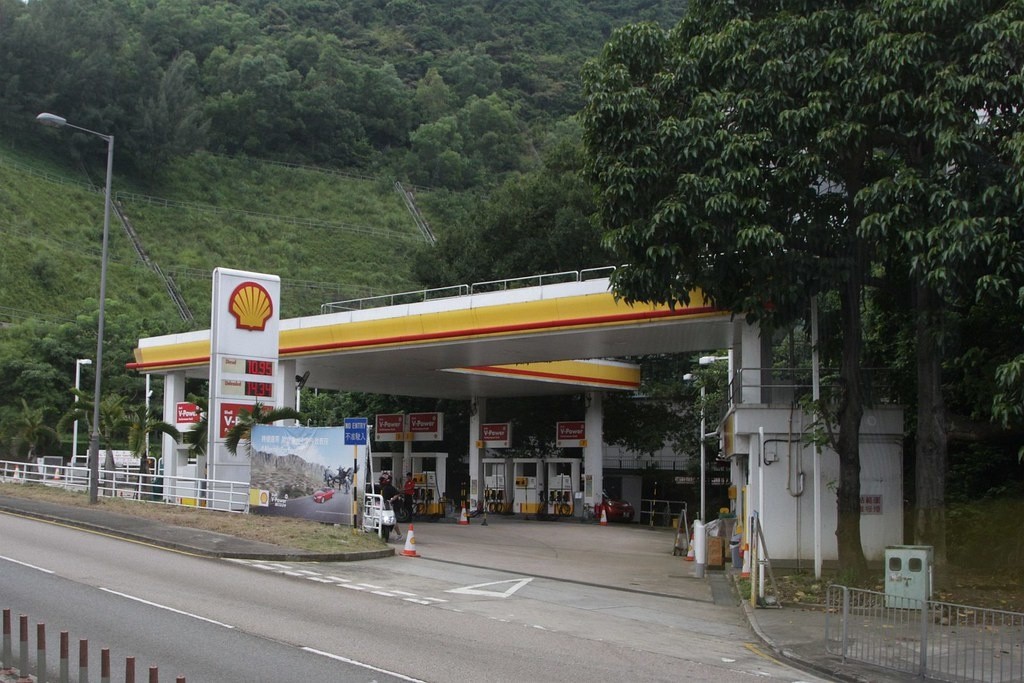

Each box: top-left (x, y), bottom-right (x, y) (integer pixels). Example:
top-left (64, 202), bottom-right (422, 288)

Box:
top-left (683, 374), bottom-right (706, 526)
top-left (699, 348), bottom-right (734, 407)
top-left (71, 358), bottom-right (93, 469)
top-left (32, 113), bottom-right (116, 502)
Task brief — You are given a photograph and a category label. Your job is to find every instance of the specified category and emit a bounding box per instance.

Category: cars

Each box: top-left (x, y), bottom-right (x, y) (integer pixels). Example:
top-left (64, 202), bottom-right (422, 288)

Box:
top-left (601, 490), bottom-right (635, 524)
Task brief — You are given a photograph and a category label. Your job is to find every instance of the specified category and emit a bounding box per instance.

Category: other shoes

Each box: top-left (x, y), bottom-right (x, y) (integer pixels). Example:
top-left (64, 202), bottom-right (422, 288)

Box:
top-left (395, 534), bottom-right (404, 541)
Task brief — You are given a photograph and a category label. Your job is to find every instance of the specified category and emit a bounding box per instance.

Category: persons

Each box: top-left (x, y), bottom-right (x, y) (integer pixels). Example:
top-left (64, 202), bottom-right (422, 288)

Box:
top-left (379, 477), bottom-right (403, 540)
top-left (403, 472), bottom-right (415, 522)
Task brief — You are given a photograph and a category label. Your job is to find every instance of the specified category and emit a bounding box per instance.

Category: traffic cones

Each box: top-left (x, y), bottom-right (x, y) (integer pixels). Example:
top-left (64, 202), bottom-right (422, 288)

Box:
top-left (741, 543), bottom-right (749, 578)
top-left (684, 532), bottom-right (694, 561)
top-left (399, 524), bottom-right (421, 558)
top-left (599, 505), bottom-right (608, 526)
top-left (458, 503), bottom-right (469, 524)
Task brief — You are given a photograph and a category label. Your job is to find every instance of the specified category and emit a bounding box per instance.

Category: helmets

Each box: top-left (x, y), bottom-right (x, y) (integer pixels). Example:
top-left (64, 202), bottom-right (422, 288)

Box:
top-left (379, 474), bottom-right (392, 486)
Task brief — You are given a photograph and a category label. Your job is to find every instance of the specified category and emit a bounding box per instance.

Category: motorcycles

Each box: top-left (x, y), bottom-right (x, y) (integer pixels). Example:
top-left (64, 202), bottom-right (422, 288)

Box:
top-left (374, 500), bottom-right (397, 543)
